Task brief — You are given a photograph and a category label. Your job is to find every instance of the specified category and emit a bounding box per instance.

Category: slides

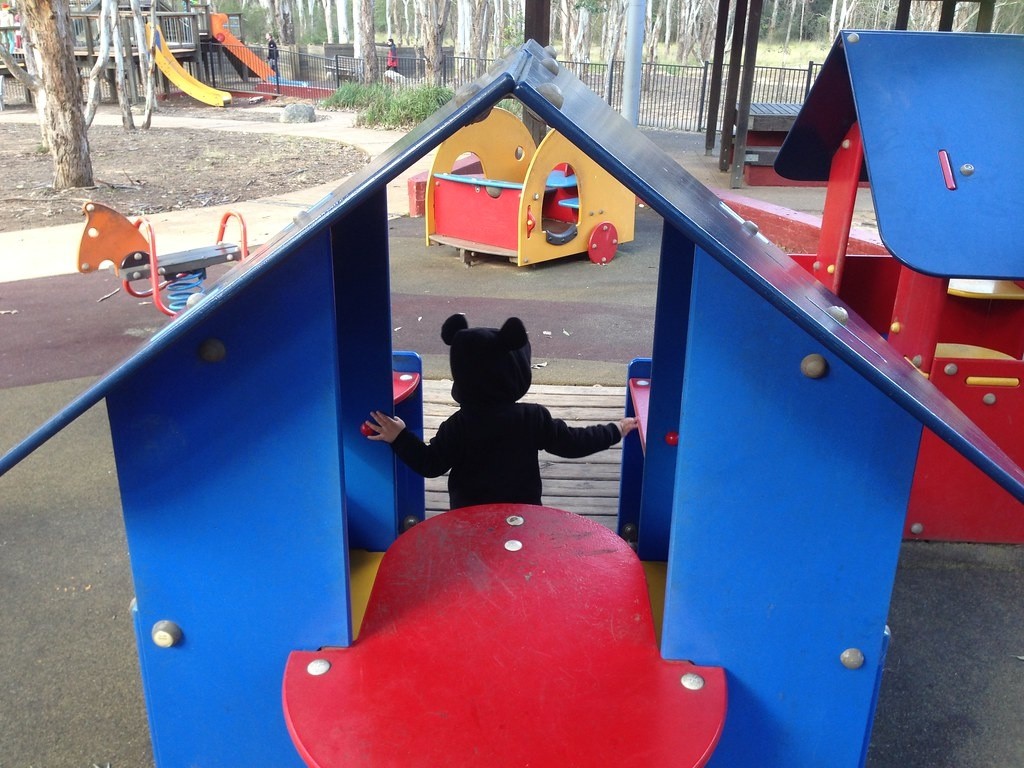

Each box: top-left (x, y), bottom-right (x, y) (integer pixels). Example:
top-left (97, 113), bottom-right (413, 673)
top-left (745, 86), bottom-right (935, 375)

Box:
top-left (146, 21), bottom-right (232, 106)
top-left (210, 12), bottom-right (310, 87)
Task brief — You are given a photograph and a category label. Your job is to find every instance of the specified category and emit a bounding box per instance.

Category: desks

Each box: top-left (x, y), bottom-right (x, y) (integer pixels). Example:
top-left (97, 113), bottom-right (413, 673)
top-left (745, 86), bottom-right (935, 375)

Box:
top-left (733, 102), bottom-right (802, 146)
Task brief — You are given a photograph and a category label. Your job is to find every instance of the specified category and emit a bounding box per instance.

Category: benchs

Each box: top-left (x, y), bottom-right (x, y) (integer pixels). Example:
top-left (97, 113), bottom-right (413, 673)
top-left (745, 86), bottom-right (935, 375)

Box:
top-left (324, 56), bottom-right (364, 83)
top-left (742, 146), bottom-right (870, 187)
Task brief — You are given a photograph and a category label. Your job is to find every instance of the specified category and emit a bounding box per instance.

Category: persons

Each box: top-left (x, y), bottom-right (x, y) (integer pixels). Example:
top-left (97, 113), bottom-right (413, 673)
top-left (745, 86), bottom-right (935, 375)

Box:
top-left (365, 314), bottom-right (641, 510)
top-left (0, 3), bottom-right (22, 55)
top-left (386, 39), bottom-right (399, 72)
top-left (265, 33), bottom-right (279, 77)
